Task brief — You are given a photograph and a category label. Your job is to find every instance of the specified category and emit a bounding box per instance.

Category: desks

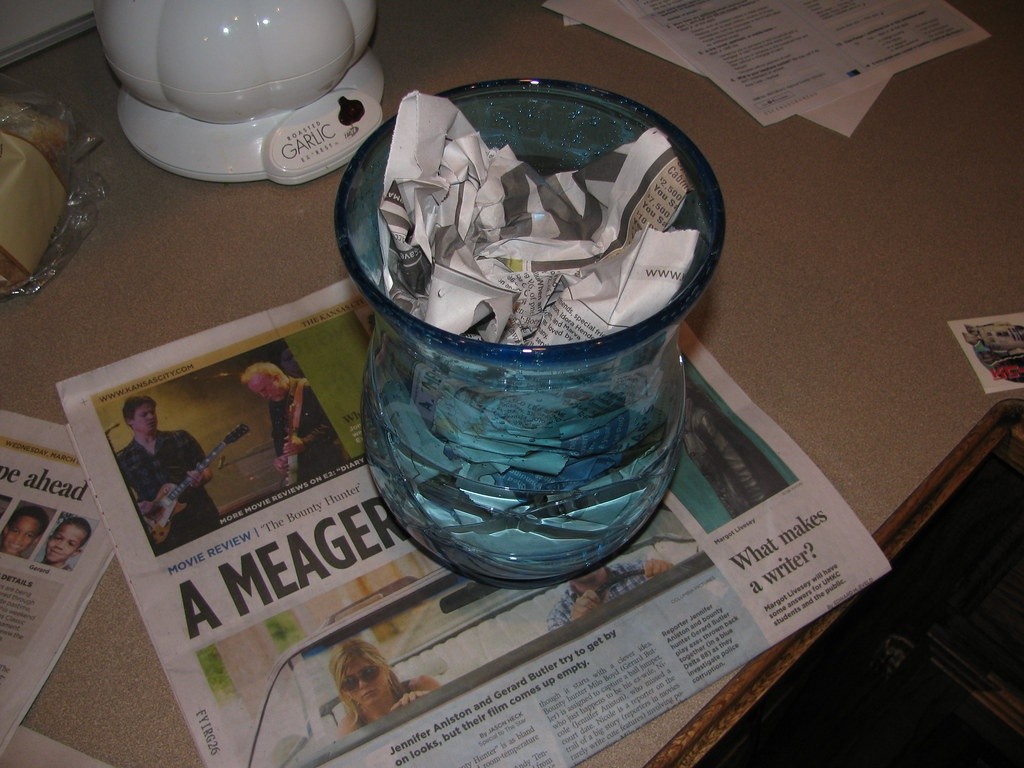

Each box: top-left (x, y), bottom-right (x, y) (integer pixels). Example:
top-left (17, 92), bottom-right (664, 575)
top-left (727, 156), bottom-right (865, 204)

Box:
top-left (0, 0), bottom-right (1024, 768)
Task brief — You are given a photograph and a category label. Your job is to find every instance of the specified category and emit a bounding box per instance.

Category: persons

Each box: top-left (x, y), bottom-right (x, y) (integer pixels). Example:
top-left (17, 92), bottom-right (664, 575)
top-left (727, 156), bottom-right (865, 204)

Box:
top-left (115, 395), bottom-right (220, 551)
top-left (328, 639), bottom-right (442, 738)
top-left (41, 517), bottom-right (92, 571)
top-left (546, 558), bottom-right (674, 633)
top-left (240, 362), bottom-right (350, 488)
top-left (0, 506), bottom-right (49, 557)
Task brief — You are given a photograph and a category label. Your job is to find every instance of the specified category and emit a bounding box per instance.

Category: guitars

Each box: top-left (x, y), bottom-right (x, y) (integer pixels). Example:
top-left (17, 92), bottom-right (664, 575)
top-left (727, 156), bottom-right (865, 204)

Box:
top-left (138, 422), bottom-right (251, 545)
top-left (283, 402), bottom-right (299, 488)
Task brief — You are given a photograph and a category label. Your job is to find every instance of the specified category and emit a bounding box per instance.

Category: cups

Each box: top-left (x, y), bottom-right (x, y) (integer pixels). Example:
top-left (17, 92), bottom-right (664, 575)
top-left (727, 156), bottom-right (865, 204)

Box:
top-left (335, 79), bottom-right (726, 590)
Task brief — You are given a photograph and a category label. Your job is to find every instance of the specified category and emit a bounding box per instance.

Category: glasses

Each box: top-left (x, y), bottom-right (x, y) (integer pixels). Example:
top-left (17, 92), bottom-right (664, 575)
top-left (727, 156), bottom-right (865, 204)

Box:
top-left (340, 665), bottom-right (379, 691)
